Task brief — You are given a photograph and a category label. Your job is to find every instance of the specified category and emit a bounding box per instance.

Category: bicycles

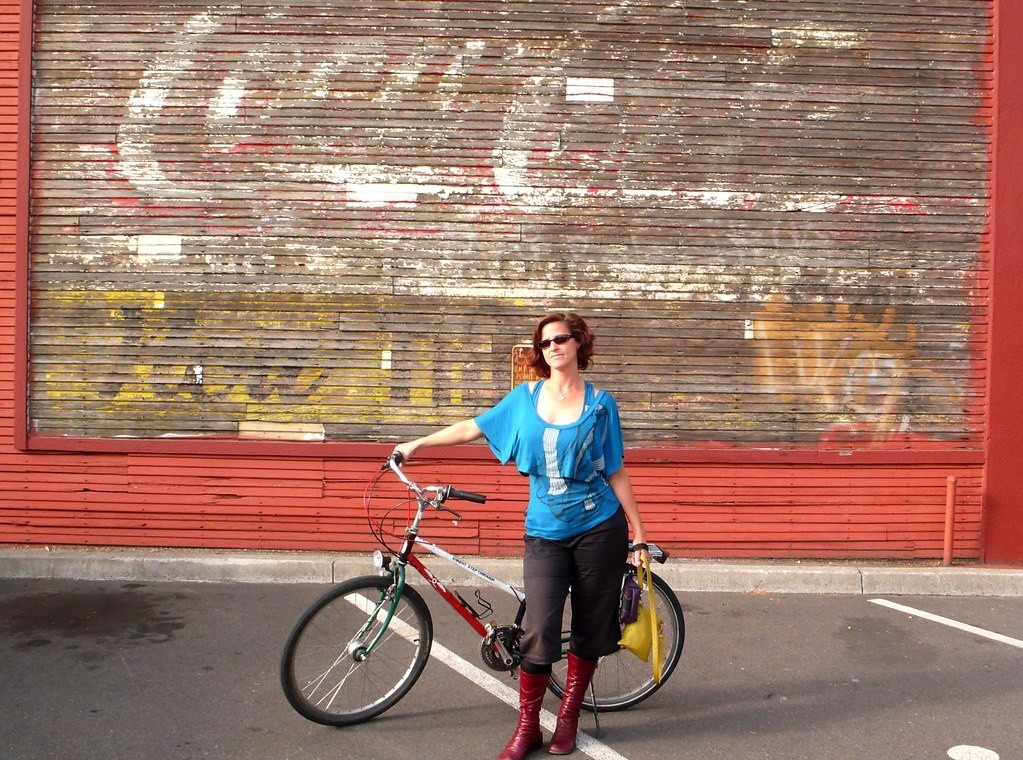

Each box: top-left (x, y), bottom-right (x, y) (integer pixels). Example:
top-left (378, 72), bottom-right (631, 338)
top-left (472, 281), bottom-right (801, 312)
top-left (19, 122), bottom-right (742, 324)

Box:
top-left (280, 448), bottom-right (689, 742)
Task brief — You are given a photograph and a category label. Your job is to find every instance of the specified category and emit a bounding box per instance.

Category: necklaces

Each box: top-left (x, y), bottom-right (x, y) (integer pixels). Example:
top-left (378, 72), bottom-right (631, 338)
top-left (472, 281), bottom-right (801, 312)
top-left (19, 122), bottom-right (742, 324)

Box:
top-left (545, 379), bottom-right (581, 401)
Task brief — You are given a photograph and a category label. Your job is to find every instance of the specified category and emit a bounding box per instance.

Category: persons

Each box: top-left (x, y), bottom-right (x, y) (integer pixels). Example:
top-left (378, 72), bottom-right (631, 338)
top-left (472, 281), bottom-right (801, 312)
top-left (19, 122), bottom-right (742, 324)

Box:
top-left (389, 311), bottom-right (653, 760)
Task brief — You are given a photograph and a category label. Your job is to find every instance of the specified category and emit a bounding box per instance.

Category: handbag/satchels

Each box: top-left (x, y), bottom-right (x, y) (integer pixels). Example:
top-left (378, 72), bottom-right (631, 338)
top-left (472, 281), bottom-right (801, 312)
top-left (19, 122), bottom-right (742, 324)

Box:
top-left (617, 602), bottom-right (662, 663)
top-left (619, 575), bottom-right (642, 624)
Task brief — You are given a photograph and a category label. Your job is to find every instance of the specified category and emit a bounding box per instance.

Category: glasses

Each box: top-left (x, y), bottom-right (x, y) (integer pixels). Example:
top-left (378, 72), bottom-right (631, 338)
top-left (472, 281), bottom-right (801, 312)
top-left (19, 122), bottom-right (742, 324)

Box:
top-left (538, 334), bottom-right (575, 349)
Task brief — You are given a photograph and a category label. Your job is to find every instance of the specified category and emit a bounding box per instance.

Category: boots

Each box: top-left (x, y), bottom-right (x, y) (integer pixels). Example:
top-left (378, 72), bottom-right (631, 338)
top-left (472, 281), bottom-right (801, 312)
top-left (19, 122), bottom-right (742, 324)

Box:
top-left (495, 668), bottom-right (552, 760)
top-left (546, 650), bottom-right (600, 755)
